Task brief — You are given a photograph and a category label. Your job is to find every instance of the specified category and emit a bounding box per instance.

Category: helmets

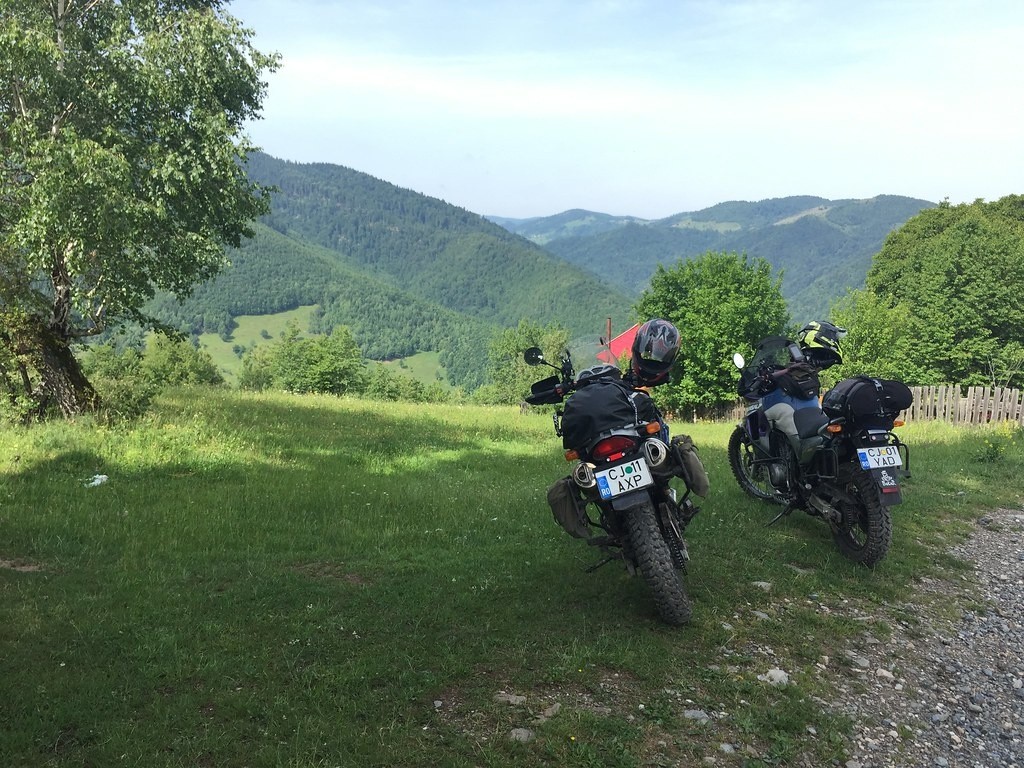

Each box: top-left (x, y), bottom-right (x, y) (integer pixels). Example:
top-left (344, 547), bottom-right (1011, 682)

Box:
top-left (630, 319), bottom-right (681, 383)
top-left (797, 320), bottom-right (845, 364)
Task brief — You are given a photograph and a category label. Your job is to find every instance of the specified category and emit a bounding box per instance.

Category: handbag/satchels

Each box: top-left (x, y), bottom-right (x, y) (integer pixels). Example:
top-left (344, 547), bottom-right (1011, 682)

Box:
top-left (822, 374), bottom-right (915, 423)
top-left (792, 371), bottom-right (818, 400)
top-left (560, 377), bottom-right (639, 450)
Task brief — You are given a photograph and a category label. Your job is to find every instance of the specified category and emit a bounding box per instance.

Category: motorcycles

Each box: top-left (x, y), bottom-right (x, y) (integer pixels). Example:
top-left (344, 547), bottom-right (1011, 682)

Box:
top-left (728, 320), bottom-right (913, 564)
top-left (523, 318), bottom-right (710, 626)
